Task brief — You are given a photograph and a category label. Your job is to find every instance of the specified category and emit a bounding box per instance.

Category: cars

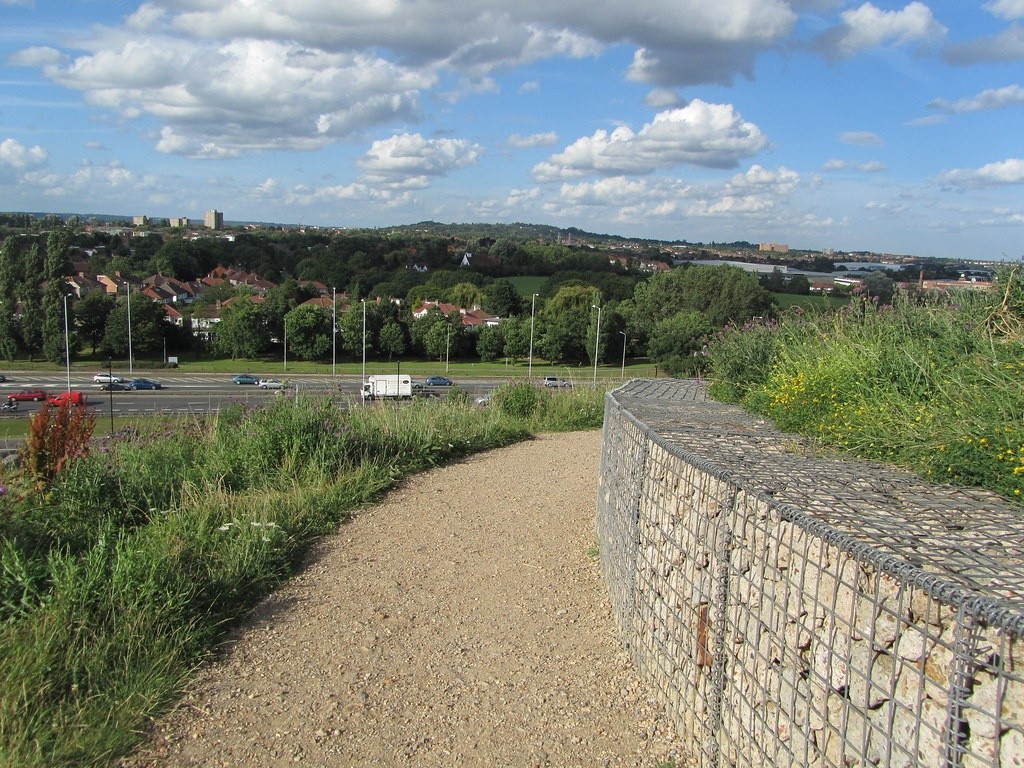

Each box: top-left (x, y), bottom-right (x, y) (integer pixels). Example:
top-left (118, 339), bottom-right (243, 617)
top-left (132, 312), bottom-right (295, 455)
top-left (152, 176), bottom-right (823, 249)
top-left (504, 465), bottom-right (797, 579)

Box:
top-left (411, 381), bottom-right (423, 389)
top-left (475, 394), bottom-right (492, 406)
top-left (232, 374), bottom-right (261, 385)
top-left (100, 384), bottom-right (131, 391)
top-left (425, 376), bottom-right (453, 386)
top-left (259, 379), bottom-right (289, 390)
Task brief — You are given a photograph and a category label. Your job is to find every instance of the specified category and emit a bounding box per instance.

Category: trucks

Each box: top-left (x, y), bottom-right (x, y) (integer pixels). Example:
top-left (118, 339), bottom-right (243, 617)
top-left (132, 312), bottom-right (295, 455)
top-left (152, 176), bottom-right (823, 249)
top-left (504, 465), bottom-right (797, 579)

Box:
top-left (359, 375), bottom-right (412, 401)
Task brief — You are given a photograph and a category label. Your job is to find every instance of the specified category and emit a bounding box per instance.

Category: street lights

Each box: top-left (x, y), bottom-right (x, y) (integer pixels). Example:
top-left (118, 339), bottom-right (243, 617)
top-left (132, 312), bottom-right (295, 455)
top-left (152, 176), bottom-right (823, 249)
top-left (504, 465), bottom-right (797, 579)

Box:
top-left (123, 281), bottom-right (133, 375)
top-left (592, 304), bottom-right (600, 389)
top-left (528, 293), bottom-right (538, 381)
top-left (332, 287), bottom-right (337, 376)
top-left (63, 293), bottom-right (73, 418)
top-left (361, 299), bottom-right (368, 411)
top-left (108, 356), bottom-right (116, 437)
top-left (397, 360), bottom-right (400, 408)
top-left (447, 323), bottom-right (452, 374)
top-left (620, 331), bottom-right (627, 378)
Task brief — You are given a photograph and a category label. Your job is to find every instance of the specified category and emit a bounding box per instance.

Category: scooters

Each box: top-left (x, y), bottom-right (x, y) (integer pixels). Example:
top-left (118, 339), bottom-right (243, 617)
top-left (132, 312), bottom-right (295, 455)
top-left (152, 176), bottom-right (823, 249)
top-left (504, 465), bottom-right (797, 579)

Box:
top-left (0, 402), bottom-right (20, 413)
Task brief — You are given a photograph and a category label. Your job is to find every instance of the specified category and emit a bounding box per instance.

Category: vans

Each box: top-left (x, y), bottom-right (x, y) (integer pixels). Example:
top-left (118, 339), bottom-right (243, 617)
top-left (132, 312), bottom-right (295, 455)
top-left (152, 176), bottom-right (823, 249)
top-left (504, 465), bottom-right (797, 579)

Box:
top-left (46, 391), bottom-right (83, 407)
top-left (543, 377), bottom-right (570, 388)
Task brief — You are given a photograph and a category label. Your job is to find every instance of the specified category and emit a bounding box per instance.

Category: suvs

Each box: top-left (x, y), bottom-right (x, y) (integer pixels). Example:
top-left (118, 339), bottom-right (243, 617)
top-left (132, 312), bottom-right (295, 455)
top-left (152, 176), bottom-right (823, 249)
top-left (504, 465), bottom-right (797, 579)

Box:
top-left (94, 373), bottom-right (124, 384)
top-left (130, 378), bottom-right (161, 391)
top-left (7, 387), bottom-right (46, 402)
